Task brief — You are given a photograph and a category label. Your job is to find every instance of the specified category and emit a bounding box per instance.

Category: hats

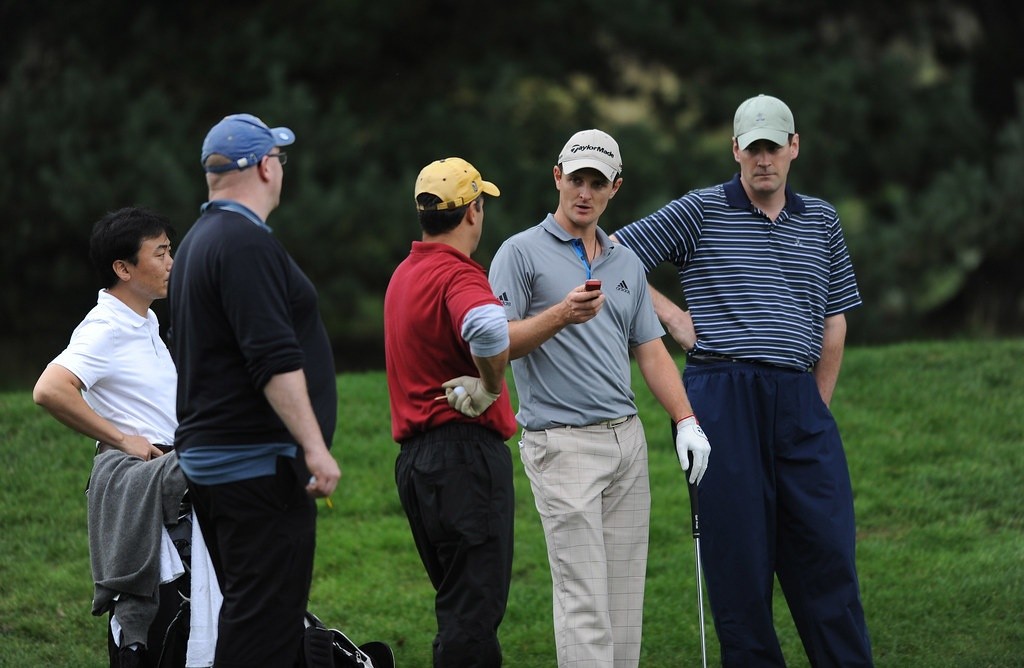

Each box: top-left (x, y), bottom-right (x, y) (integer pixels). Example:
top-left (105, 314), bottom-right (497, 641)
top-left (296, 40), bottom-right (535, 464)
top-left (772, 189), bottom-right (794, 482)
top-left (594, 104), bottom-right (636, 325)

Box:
top-left (558, 129), bottom-right (623, 183)
top-left (201, 113), bottom-right (295, 173)
top-left (414, 157), bottom-right (500, 211)
top-left (733, 94), bottom-right (795, 152)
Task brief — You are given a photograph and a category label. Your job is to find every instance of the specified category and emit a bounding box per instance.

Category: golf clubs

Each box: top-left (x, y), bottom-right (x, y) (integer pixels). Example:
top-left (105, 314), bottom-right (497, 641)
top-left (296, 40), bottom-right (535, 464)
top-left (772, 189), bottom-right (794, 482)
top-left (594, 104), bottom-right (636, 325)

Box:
top-left (688, 474), bottom-right (706, 668)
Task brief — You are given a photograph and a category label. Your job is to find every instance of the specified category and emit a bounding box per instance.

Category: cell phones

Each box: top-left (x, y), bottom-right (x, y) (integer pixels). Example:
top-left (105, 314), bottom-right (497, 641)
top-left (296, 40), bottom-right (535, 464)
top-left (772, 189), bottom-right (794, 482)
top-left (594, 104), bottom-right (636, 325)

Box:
top-left (586, 279), bottom-right (601, 300)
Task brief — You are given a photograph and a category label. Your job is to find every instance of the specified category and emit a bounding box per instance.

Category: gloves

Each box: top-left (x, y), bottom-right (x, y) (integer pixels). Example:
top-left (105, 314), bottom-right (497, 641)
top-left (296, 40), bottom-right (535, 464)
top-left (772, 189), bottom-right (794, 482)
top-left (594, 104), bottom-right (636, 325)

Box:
top-left (676, 414), bottom-right (711, 486)
top-left (441, 375), bottom-right (501, 418)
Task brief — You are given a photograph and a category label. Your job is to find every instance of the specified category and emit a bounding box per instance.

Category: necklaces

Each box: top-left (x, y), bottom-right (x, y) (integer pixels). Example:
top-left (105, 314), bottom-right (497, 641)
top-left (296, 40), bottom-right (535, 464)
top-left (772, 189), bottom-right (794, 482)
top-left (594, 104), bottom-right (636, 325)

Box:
top-left (593, 239), bottom-right (596, 258)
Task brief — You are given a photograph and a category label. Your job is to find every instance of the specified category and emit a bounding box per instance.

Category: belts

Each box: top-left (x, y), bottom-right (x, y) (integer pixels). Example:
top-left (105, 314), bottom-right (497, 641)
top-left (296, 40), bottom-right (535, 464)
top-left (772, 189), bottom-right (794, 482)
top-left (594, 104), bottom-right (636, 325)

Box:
top-left (587, 414), bottom-right (635, 429)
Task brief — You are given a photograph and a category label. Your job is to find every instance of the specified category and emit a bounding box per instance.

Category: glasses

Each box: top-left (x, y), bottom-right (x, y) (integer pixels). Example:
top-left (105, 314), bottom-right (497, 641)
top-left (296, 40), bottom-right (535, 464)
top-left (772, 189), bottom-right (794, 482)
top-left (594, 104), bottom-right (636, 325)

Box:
top-left (268, 152), bottom-right (288, 165)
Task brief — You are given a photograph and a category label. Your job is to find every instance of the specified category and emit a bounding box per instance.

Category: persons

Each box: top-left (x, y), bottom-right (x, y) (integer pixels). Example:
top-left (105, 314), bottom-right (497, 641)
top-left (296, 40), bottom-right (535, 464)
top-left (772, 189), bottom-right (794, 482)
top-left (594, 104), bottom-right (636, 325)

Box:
top-left (169, 112), bottom-right (339, 668)
top-left (35, 203), bottom-right (190, 668)
top-left (384, 159), bottom-right (511, 668)
top-left (606, 88), bottom-right (864, 668)
top-left (488, 128), bottom-right (711, 668)
